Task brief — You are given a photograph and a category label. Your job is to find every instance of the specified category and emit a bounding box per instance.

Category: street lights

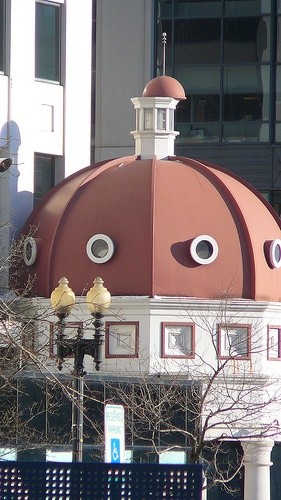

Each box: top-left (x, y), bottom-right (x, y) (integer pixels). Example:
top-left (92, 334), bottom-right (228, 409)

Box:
top-left (47, 276), bottom-right (111, 464)
